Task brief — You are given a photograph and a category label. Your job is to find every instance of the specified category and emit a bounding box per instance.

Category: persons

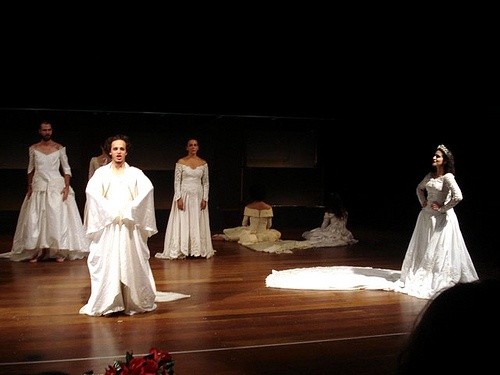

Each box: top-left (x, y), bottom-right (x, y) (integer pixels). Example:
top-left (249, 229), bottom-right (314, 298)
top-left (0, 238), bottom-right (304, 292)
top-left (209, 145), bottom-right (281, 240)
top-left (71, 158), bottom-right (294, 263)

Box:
top-left (212, 185), bottom-right (293, 254)
top-left (78, 135), bottom-right (158, 317)
top-left (0, 122), bottom-right (90, 262)
top-left (302, 192), bottom-right (359, 247)
top-left (88, 138), bottom-right (112, 180)
top-left (155, 138), bottom-right (217, 260)
top-left (396, 280), bottom-right (500, 375)
top-left (401, 145), bottom-right (479, 300)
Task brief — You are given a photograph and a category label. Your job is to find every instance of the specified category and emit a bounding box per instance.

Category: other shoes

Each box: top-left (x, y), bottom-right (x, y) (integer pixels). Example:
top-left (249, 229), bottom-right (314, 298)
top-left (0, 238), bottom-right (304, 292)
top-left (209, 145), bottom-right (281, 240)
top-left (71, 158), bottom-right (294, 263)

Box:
top-left (29, 251), bottom-right (43, 262)
top-left (57, 255), bottom-right (65, 262)
